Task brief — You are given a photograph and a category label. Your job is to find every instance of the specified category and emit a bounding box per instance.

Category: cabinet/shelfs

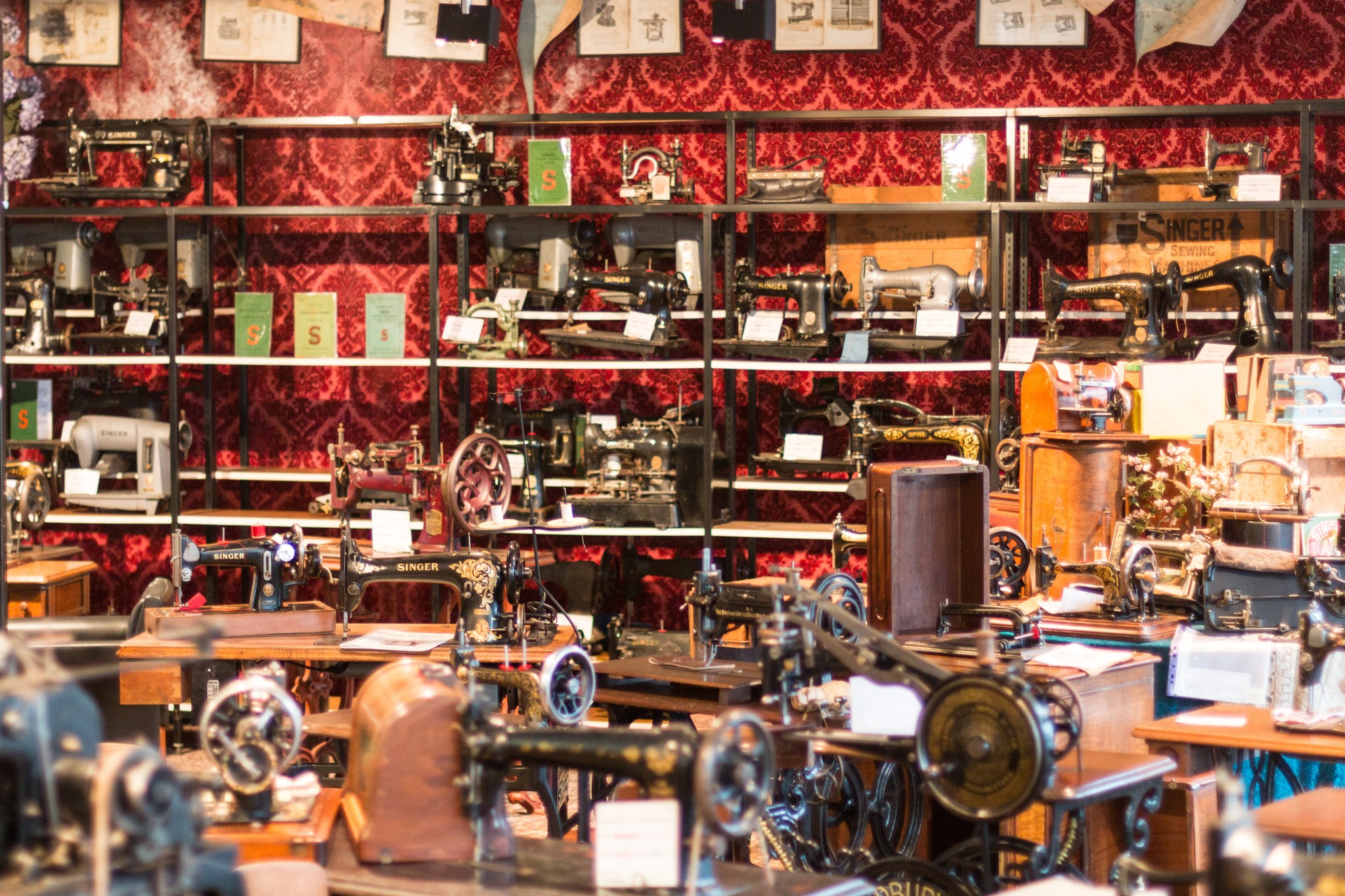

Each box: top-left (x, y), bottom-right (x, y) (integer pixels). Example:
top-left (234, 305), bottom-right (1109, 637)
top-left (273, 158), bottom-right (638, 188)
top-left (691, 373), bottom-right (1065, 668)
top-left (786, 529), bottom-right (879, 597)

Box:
top-left (0, 190), bottom-right (1345, 555)
